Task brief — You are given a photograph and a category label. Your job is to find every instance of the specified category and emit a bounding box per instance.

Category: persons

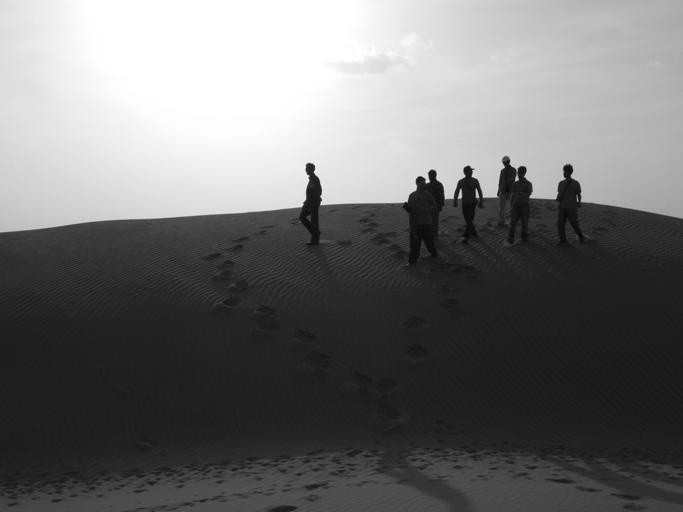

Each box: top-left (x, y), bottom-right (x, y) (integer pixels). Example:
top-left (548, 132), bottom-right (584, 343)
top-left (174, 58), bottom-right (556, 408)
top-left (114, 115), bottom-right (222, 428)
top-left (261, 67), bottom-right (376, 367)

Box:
top-left (403, 156), bottom-right (583, 263)
top-left (299, 163), bottom-right (322, 246)
